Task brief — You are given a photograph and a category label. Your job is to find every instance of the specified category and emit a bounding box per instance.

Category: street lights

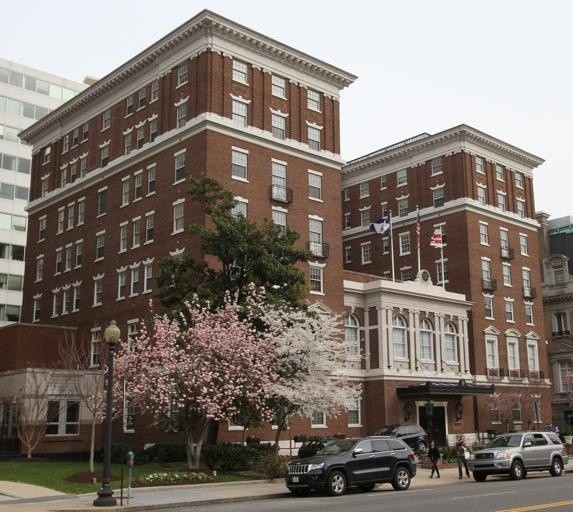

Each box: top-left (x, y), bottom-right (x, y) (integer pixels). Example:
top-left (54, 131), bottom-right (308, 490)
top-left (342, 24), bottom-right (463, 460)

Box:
top-left (102, 319), bottom-right (120, 488)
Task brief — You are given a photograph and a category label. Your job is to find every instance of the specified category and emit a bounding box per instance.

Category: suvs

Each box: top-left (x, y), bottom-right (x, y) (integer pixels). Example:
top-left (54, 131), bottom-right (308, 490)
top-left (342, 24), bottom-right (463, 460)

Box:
top-left (374, 424), bottom-right (429, 454)
top-left (467, 431), bottom-right (568, 482)
top-left (286, 434), bottom-right (417, 494)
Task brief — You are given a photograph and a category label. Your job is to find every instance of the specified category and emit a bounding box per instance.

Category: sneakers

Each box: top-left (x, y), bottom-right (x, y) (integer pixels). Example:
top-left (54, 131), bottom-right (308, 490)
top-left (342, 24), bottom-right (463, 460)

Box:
top-left (459, 475), bottom-right (470, 479)
top-left (430, 475), bottom-right (441, 478)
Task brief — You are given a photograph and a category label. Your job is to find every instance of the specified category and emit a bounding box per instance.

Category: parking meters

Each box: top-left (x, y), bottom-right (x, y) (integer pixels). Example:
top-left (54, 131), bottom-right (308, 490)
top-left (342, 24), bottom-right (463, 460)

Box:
top-left (125, 451), bottom-right (135, 504)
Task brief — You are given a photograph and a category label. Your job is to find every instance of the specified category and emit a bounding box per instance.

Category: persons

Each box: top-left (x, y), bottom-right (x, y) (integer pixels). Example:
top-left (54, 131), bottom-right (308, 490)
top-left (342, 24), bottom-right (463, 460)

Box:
top-left (456, 438), bottom-right (472, 479)
top-left (555, 426), bottom-right (566, 443)
top-left (428, 440), bottom-right (441, 478)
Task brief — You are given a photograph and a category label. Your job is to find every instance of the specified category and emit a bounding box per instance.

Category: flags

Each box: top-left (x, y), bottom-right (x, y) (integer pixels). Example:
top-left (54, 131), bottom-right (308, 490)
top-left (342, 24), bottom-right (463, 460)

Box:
top-left (417, 213), bottom-right (422, 248)
top-left (369, 215), bottom-right (390, 237)
top-left (429, 228), bottom-right (442, 248)
